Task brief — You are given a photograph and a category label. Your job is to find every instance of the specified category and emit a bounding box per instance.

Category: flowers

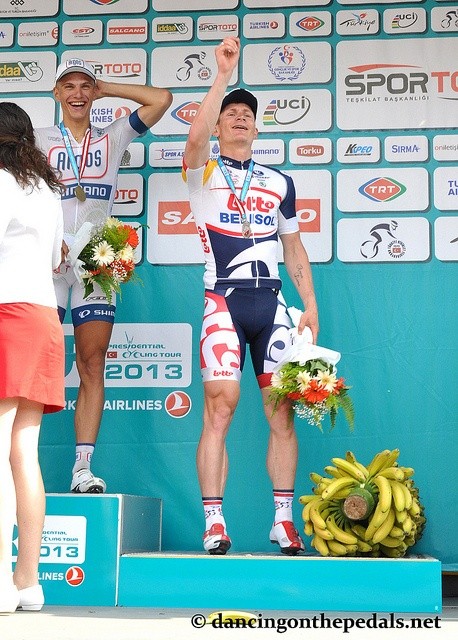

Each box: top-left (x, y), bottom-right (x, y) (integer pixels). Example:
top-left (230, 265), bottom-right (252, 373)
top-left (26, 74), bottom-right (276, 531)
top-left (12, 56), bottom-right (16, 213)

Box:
top-left (81, 211), bottom-right (150, 308)
top-left (261, 361), bottom-right (355, 434)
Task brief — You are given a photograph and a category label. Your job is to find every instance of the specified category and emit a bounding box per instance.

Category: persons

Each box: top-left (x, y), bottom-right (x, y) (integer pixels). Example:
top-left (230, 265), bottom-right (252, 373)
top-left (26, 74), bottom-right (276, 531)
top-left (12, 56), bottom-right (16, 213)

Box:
top-left (181, 35), bottom-right (319, 555)
top-left (0, 101), bottom-right (66, 613)
top-left (29, 59), bottom-right (173, 492)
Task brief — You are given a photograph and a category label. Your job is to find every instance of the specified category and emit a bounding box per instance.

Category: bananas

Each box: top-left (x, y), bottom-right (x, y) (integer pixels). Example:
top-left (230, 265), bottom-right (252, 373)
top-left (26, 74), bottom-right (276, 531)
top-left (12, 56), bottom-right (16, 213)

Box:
top-left (299, 447), bottom-right (426, 559)
top-left (198, 610), bottom-right (258, 626)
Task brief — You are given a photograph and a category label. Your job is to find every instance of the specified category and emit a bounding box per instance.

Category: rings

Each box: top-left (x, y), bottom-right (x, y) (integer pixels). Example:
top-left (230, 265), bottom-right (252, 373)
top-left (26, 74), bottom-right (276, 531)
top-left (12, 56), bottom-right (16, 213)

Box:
top-left (220, 40), bottom-right (224, 46)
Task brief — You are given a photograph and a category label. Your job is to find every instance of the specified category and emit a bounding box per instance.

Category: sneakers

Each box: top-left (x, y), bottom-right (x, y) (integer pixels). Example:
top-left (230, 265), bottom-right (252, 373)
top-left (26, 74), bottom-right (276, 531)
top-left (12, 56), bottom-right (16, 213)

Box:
top-left (204, 524), bottom-right (231, 556)
top-left (71, 468), bottom-right (107, 493)
top-left (18, 583), bottom-right (44, 612)
top-left (0, 584), bottom-right (21, 612)
top-left (268, 521), bottom-right (302, 551)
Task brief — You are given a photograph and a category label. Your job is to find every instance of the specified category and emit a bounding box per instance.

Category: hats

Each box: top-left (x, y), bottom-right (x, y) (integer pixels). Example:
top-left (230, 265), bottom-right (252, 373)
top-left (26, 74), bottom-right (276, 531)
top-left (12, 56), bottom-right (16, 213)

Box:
top-left (55, 58), bottom-right (97, 86)
top-left (221, 88), bottom-right (257, 119)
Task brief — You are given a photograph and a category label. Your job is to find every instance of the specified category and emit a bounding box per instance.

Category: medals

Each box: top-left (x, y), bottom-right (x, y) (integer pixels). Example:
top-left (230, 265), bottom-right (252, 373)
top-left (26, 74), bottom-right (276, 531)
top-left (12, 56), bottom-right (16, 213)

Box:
top-left (217, 156), bottom-right (255, 238)
top-left (58, 121), bottom-right (91, 201)
